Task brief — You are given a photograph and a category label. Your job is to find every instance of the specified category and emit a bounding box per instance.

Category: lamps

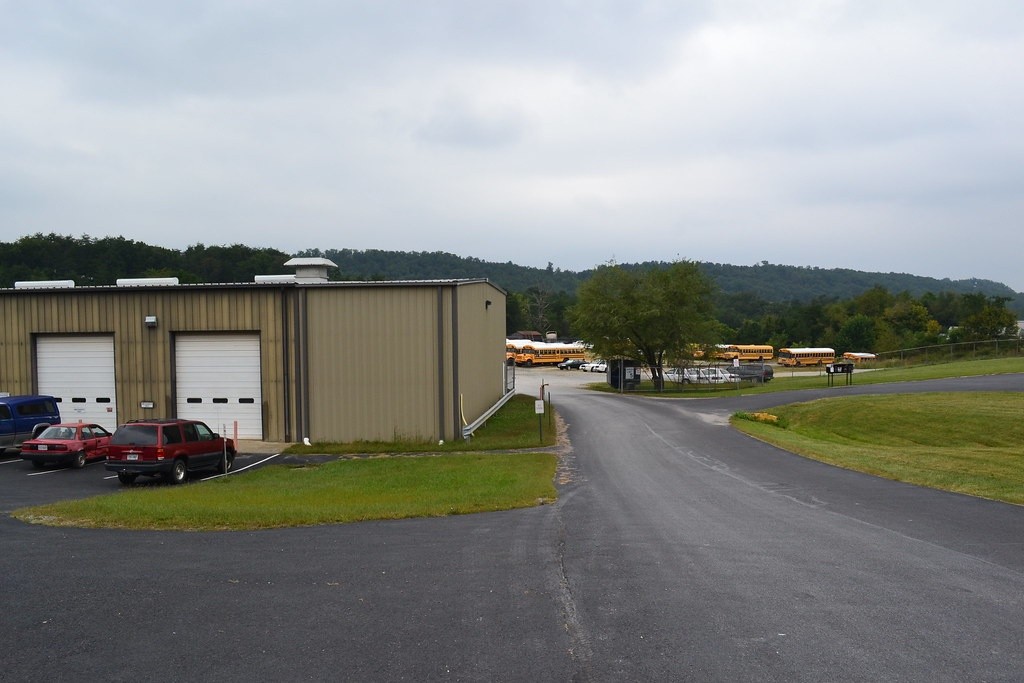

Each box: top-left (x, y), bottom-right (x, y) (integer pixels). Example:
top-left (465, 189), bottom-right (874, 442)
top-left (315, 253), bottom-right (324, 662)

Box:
top-left (145, 316), bottom-right (157, 327)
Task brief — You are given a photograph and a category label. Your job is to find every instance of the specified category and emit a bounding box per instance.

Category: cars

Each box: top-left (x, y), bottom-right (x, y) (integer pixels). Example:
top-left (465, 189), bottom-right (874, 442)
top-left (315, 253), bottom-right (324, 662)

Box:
top-left (557, 358), bottom-right (588, 371)
top-left (641, 366), bottom-right (742, 385)
top-left (19, 422), bottom-right (113, 470)
top-left (579, 358), bottom-right (609, 374)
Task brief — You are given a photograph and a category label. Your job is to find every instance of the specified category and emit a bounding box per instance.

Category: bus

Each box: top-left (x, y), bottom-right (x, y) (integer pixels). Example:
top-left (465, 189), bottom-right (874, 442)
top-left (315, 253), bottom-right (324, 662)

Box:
top-left (777, 347), bottom-right (836, 368)
top-left (675, 342), bottom-right (733, 361)
top-left (506, 338), bottom-right (586, 368)
top-left (724, 344), bottom-right (774, 361)
top-left (843, 352), bottom-right (879, 367)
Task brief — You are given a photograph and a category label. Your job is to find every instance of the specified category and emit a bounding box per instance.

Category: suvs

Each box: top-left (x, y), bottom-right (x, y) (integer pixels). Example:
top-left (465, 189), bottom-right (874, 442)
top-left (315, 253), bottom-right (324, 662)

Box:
top-left (726, 363), bottom-right (775, 383)
top-left (104, 418), bottom-right (237, 486)
top-left (0, 393), bottom-right (62, 454)
